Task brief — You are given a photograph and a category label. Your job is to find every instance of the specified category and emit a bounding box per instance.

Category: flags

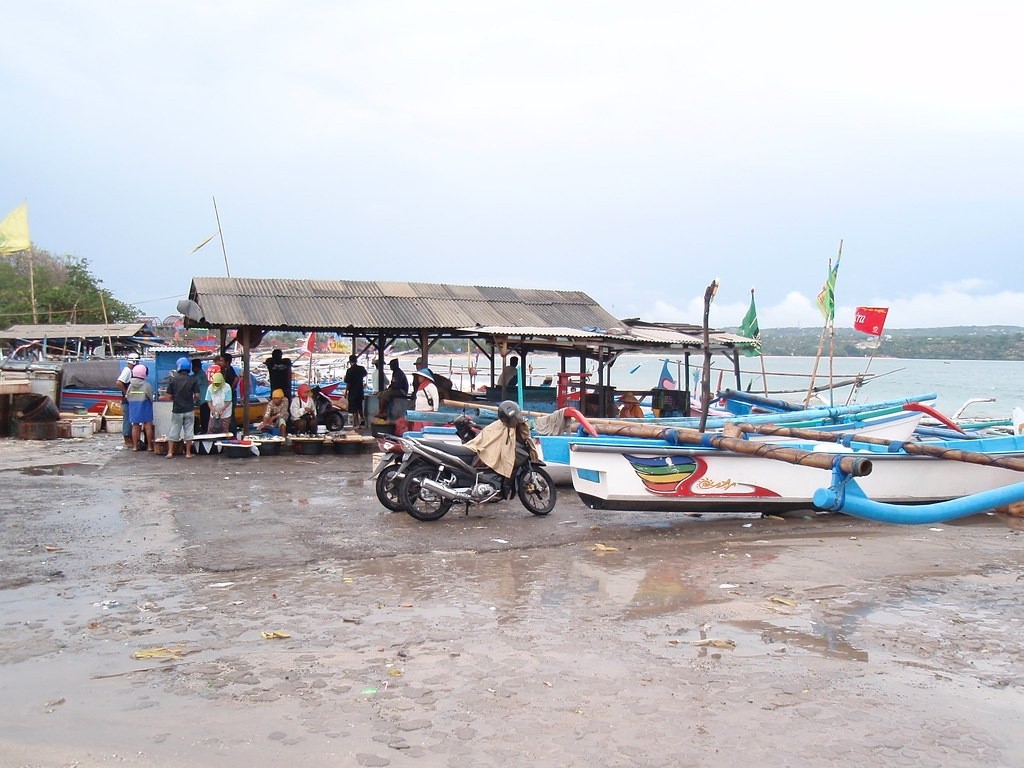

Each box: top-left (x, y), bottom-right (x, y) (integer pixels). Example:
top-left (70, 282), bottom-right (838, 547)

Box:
top-left (855, 306), bottom-right (888, 336)
top-left (815, 262), bottom-right (839, 318)
top-left (741, 298), bottom-right (761, 357)
top-left (0, 202), bottom-right (30, 254)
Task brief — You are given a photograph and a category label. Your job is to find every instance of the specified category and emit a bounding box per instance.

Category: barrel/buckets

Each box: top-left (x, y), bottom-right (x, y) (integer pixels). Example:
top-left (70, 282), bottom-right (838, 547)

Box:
top-left (372, 453), bottom-right (395, 479)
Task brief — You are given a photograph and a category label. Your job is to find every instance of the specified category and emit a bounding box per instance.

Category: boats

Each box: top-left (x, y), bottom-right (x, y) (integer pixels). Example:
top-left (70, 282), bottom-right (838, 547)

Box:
top-left (393, 386), bottom-right (940, 487)
top-left (567, 396), bottom-right (1022, 526)
top-left (57, 339), bottom-right (391, 418)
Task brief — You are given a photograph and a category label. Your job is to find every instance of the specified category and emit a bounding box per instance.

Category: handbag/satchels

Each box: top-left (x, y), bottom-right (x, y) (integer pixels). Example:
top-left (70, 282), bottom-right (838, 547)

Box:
top-left (428, 399), bottom-right (433, 405)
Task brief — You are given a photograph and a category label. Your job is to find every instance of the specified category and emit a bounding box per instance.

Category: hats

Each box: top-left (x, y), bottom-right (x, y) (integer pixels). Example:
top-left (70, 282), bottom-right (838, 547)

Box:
top-left (387, 358), bottom-right (398, 365)
top-left (413, 357), bottom-right (422, 365)
top-left (372, 359), bottom-right (386, 365)
top-left (616, 392), bottom-right (639, 402)
top-left (544, 376), bottom-right (552, 380)
top-left (412, 368), bottom-right (435, 382)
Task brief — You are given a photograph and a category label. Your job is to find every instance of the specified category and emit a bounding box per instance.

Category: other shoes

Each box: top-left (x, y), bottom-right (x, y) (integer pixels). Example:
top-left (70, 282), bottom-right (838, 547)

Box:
top-left (165, 453), bottom-right (172, 458)
top-left (186, 454), bottom-right (196, 459)
top-left (350, 426), bottom-right (361, 430)
top-left (375, 415), bottom-right (387, 420)
top-left (123, 440), bottom-right (154, 452)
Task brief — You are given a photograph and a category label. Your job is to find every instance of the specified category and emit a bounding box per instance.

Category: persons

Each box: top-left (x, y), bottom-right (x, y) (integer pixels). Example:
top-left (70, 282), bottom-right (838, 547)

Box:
top-left (617, 392), bottom-right (644, 418)
top-left (205, 373), bottom-right (233, 432)
top-left (411, 368), bottom-right (440, 429)
top-left (343, 355), bottom-right (367, 429)
top-left (257, 389), bottom-right (289, 437)
top-left (166, 357), bottom-right (200, 459)
top-left (116, 354), bottom-right (141, 447)
top-left (266, 348), bottom-right (292, 407)
top-left (190, 359), bottom-right (211, 433)
top-left (375, 359), bottom-right (409, 419)
top-left (411, 357), bottom-right (423, 399)
top-left (498, 356), bottom-right (518, 386)
top-left (220, 354), bottom-right (239, 434)
top-left (125, 365), bottom-right (154, 451)
top-left (290, 384), bottom-right (318, 434)
top-left (539, 376), bottom-right (552, 387)
top-left (371, 360), bottom-right (390, 389)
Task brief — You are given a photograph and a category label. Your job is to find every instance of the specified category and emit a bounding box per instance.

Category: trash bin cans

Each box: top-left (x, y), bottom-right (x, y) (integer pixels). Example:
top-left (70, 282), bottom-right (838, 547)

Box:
top-left (579, 393), bottom-right (600, 418)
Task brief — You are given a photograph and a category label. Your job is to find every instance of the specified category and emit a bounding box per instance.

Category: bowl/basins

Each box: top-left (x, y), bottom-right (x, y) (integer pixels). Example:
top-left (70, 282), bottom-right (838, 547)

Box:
top-left (334, 443), bottom-right (361, 455)
top-left (195, 440), bottom-right (281, 457)
top-left (293, 440), bottom-right (323, 454)
top-left (371, 423), bottom-right (396, 438)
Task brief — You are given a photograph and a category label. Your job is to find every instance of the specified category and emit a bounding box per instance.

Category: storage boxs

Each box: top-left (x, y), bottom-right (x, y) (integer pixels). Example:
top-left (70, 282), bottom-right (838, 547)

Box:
top-left (56, 420), bottom-right (71, 438)
top-left (105, 416), bottom-right (124, 433)
top-left (0, 380), bottom-right (33, 394)
top-left (60, 415), bottom-right (102, 433)
top-left (70, 421), bottom-right (93, 438)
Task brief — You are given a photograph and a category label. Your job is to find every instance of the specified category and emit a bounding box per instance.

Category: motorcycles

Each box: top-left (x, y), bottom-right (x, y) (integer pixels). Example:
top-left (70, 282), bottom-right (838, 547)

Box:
top-left (367, 403), bottom-right (511, 513)
top-left (292, 379), bottom-right (345, 432)
top-left (388, 400), bottom-right (557, 522)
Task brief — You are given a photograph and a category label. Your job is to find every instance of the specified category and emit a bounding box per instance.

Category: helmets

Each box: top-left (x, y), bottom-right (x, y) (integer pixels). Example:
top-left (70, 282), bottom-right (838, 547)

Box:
top-left (176, 357), bottom-right (191, 373)
top-left (132, 364), bottom-right (147, 379)
top-left (498, 400), bottom-right (521, 427)
top-left (127, 352), bottom-right (140, 366)
